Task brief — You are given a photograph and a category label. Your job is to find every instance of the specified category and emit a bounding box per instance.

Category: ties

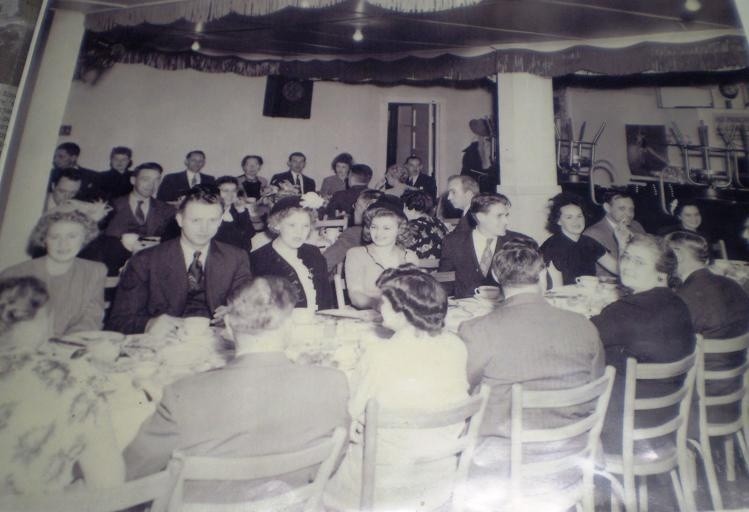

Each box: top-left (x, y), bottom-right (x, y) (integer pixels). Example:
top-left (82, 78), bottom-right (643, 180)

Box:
top-left (188, 252), bottom-right (203, 282)
top-left (295, 174), bottom-right (302, 193)
top-left (409, 177), bottom-right (414, 186)
top-left (190, 174), bottom-right (196, 185)
top-left (135, 199), bottom-right (145, 224)
top-left (480, 239), bottom-right (493, 269)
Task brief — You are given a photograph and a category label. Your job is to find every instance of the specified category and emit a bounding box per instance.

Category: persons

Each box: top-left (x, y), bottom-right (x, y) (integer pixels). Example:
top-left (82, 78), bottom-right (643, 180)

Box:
top-left (454, 193), bottom-right (553, 300)
top-left (212, 175), bottom-right (256, 240)
top-left (324, 262), bottom-right (470, 512)
top-left (42, 167), bottom-right (83, 209)
top-left (103, 184), bottom-right (253, 335)
top-left (249, 196), bottom-right (334, 312)
top-left (323, 189), bottom-right (386, 279)
top-left (104, 161), bottom-right (180, 239)
top-left (324, 163), bottom-right (374, 219)
top-left (455, 236), bottom-right (607, 495)
top-left (585, 189), bottom-right (647, 277)
top-left (49, 142), bottom-right (99, 203)
top-left (344, 208), bottom-right (420, 311)
top-left (235, 155), bottom-right (268, 203)
top-left (156, 150), bottom-right (216, 203)
top-left (0, 274), bottom-right (127, 512)
top-left (651, 201), bottom-right (712, 256)
top-left (1, 210), bottom-right (109, 339)
top-left (396, 188), bottom-right (450, 274)
top-left (438, 174), bottom-right (480, 294)
top-left (539, 194), bottom-right (620, 291)
top-left (589, 231), bottom-right (697, 487)
top-left (383, 165), bottom-right (421, 199)
top-left (270, 152), bottom-right (316, 196)
top-left (401, 155), bottom-right (437, 205)
top-left (665, 228), bottom-right (749, 471)
top-left (121, 273), bottom-right (351, 512)
top-left (320, 153), bottom-right (354, 195)
top-left (99, 146), bottom-right (135, 203)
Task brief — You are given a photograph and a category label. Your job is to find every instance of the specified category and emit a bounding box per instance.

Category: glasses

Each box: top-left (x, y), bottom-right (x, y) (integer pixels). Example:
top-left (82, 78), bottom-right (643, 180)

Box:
top-left (221, 187), bottom-right (239, 194)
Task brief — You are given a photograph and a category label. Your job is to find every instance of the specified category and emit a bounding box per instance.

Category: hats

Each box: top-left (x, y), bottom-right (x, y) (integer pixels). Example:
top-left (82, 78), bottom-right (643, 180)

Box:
top-left (112, 146), bottom-right (133, 157)
top-left (270, 191), bottom-right (325, 215)
top-left (336, 153), bottom-right (353, 164)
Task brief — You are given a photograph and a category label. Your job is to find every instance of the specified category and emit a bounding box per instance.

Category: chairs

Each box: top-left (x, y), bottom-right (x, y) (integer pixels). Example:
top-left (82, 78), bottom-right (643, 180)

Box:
top-left (512, 365), bottom-right (618, 512)
top-left (362, 383), bottom-right (491, 512)
top-left (169, 429), bottom-right (347, 512)
top-left (602, 336), bottom-right (700, 512)
top-left (693, 330), bottom-right (749, 487)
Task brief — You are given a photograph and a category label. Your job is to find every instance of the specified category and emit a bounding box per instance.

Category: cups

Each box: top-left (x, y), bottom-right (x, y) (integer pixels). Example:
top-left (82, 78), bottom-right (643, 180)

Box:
top-left (183, 316), bottom-right (210, 338)
top-left (474, 286), bottom-right (500, 299)
top-left (576, 276), bottom-right (598, 288)
top-left (292, 308), bottom-right (314, 324)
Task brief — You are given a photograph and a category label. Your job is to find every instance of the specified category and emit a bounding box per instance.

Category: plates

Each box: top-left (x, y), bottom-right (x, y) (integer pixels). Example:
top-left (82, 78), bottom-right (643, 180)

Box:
top-left (76, 330), bottom-right (127, 344)
